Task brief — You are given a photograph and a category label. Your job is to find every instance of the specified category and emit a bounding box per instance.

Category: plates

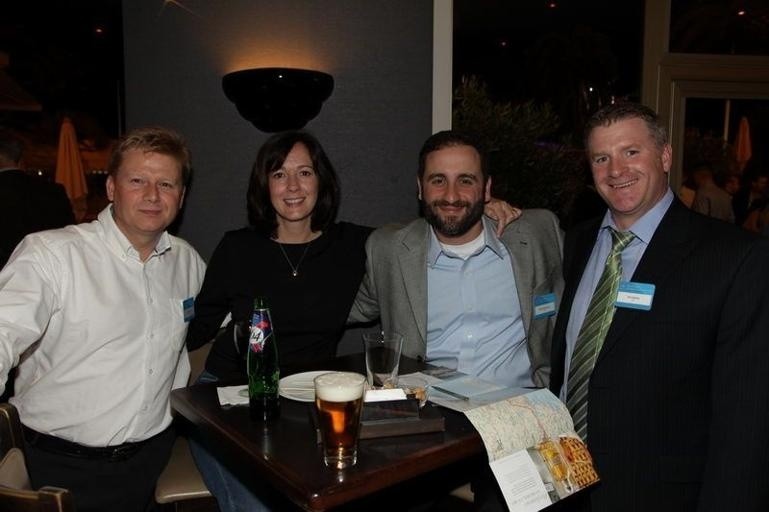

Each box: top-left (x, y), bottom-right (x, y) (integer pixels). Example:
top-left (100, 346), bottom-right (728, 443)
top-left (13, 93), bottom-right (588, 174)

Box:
top-left (276, 371), bottom-right (341, 404)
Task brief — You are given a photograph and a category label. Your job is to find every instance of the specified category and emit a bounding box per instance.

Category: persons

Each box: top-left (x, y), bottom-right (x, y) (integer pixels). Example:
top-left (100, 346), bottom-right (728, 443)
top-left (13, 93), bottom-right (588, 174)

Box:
top-left (0, 128), bottom-right (209, 510)
top-left (547, 99), bottom-right (767, 511)
top-left (0, 129), bottom-right (75, 271)
top-left (186, 130), bottom-right (523, 511)
top-left (679, 118), bottom-right (768, 235)
top-left (334, 131), bottom-right (569, 512)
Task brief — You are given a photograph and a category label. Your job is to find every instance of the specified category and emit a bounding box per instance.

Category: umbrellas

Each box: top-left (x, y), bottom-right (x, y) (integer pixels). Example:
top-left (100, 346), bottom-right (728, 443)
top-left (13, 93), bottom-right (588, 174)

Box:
top-left (57, 113), bottom-right (88, 224)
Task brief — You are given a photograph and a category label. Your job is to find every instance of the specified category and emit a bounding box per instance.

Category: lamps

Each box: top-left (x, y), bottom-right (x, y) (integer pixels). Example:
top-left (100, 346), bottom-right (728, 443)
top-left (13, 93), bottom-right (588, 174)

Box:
top-left (218, 68), bottom-right (334, 134)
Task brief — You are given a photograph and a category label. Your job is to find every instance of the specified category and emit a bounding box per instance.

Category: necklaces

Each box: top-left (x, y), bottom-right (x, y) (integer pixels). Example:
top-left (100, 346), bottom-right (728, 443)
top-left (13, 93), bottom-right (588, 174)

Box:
top-left (275, 227), bottom-right (315, 278)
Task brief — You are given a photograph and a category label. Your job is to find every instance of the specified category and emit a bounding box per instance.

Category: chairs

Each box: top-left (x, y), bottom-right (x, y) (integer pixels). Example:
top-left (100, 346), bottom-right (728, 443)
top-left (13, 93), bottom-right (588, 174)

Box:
top-left (1, 403), bottom-right (73, 512)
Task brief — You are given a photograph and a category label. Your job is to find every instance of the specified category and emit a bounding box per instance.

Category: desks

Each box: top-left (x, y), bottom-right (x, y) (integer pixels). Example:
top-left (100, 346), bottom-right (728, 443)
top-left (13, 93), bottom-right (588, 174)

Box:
top-left (166, 347), bottom-right (547, 509)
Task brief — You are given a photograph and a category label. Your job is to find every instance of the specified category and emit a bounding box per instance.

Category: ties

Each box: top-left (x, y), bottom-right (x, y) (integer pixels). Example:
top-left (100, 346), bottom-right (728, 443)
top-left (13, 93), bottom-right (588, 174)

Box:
top-left (567, 228), bottom-right (634, 446)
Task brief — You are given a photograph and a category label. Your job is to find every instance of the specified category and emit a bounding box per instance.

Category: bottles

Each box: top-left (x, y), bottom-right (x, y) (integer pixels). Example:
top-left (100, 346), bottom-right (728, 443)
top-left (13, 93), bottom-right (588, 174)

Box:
top-left (243, 292), bottom-right (282, 425)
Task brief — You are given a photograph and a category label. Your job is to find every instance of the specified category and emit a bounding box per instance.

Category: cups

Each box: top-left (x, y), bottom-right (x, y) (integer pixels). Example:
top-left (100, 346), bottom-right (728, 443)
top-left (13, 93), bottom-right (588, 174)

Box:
top-left (311, 371), bottom-right (367, 469)
top-left (398, 376), bottom-right (429, 409)
top-left (362, 328), bottom-right (406, 390)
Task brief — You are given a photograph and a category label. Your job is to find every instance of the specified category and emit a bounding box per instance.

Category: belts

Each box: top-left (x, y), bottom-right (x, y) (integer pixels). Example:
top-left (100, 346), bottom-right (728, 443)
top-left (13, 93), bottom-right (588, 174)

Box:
top-left (60, 441), bottom-right (146, 463)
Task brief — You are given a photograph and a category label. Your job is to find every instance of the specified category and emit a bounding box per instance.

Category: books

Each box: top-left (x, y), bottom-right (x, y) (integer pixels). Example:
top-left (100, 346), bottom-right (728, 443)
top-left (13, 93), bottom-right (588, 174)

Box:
top-left (372, 365), bottom-right (602, 512)
